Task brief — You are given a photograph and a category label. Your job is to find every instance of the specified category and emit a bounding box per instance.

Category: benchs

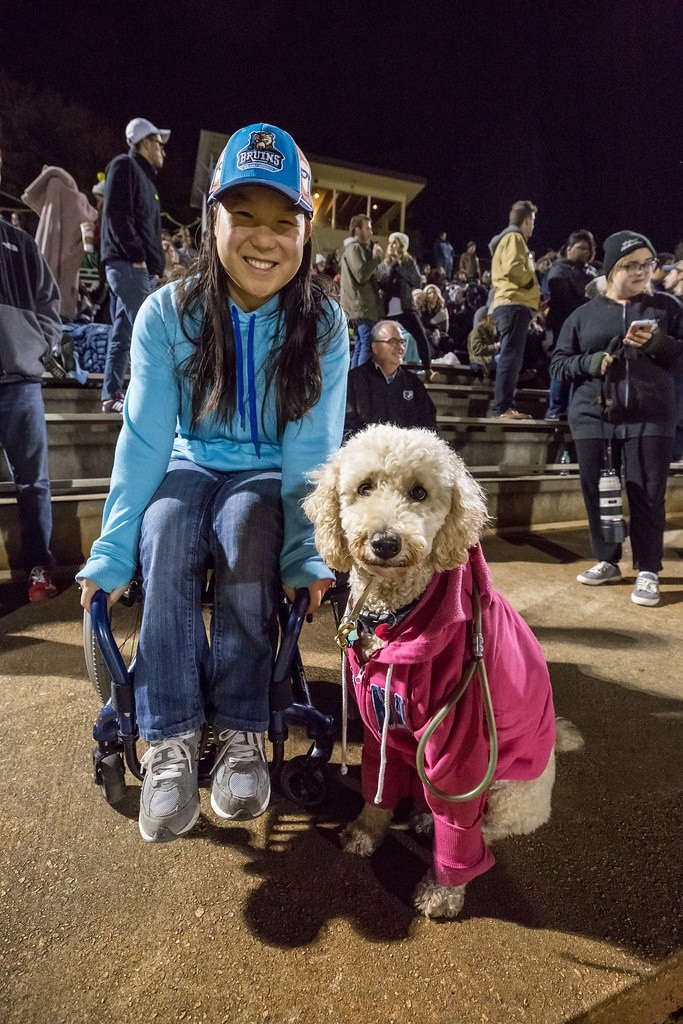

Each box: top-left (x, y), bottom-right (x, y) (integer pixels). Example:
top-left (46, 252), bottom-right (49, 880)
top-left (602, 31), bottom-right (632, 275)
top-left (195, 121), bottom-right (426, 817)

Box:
top-left (0, 362), bottom-right (683, 495)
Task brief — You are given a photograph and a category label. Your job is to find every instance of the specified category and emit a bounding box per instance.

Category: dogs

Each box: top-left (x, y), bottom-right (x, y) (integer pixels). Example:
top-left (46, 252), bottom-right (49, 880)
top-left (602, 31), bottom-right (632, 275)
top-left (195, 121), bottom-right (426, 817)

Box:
top-left (301, 420), bottom-right (557, 917)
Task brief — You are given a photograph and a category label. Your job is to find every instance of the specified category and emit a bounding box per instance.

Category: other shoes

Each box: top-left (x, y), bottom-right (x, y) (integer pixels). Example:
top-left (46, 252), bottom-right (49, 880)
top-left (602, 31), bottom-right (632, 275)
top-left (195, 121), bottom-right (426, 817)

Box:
top-left (494, 408), bottom-right (532, 421)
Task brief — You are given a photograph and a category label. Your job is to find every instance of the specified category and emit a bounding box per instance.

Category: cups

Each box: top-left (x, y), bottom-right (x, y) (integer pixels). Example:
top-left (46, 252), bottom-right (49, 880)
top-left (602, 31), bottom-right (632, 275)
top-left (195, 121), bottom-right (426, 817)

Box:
top-left (80, 222), bottom-right (93, 251)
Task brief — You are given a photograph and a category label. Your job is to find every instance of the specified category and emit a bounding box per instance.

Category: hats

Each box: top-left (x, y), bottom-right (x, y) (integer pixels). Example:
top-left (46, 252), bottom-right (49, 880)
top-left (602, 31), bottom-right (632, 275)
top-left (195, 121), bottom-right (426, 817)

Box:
top-left (126, 118), bottom-right (171, 148)
top-left (602, 232), bottom-right (656, 280)
top-left (92, 181), bottom-right (106, 195)
top-left (206, 124), bottom-right (312, 211)
top-left (393, 232), bottom-right (409, 251)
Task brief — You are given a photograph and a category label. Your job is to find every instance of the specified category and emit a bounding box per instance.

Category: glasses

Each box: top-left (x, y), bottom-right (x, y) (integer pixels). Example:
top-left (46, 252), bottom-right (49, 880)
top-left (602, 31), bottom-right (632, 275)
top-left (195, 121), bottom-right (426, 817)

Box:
top-left (375, 336), bottom-right (408, 348)
top-left (151, 138), bottom-right (165, 151)
top-left (618, 259), bottom-right (659, 274)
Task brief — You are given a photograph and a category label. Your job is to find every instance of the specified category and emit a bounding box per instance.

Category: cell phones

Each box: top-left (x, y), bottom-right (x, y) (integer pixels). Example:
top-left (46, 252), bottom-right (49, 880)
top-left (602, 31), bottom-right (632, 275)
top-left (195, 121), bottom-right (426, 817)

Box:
top-left (623, 320), bottom-right (654, 340)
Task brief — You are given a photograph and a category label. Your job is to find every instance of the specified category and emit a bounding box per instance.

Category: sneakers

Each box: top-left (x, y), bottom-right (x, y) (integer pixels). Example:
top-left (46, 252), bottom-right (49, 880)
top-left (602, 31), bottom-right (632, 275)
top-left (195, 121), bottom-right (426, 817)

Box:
top-left (102, 399), bottom-right (126, 413)
top-left (629, 570), bottom-right (661, 606)
top-left (209, 723), bottom-right (272, 820)
top-left (577, 561), bottom-right (624, 585)
top-left (27, 560), bottom-right (59, 603)
top-left (138, 726), bottom-right (204, 839)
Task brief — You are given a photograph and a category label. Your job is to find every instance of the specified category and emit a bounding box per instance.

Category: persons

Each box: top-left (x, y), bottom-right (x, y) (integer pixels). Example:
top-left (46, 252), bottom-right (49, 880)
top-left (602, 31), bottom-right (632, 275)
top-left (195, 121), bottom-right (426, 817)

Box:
top-left (0, 219), bottom-right (64, 603)
top-left (92, 117), bottom-right (201, 411)
top-left (548, 230), bottom-right (683, 606)
top-left (75, 123), bottom-right (351, 842)
top-left (317, 200), bottom-right (683, 476)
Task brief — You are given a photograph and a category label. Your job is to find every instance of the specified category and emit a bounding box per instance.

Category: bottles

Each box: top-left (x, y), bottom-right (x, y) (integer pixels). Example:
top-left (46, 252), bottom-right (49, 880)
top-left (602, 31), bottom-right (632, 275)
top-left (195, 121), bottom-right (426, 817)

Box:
top-left (560, 451), bottom-right (570, 475)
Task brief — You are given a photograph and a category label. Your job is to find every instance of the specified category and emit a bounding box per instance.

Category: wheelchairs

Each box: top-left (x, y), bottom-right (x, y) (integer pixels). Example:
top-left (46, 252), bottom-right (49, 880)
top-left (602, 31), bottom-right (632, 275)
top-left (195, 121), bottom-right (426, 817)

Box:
top-left (79, 557), bottom-right (351, 810)
